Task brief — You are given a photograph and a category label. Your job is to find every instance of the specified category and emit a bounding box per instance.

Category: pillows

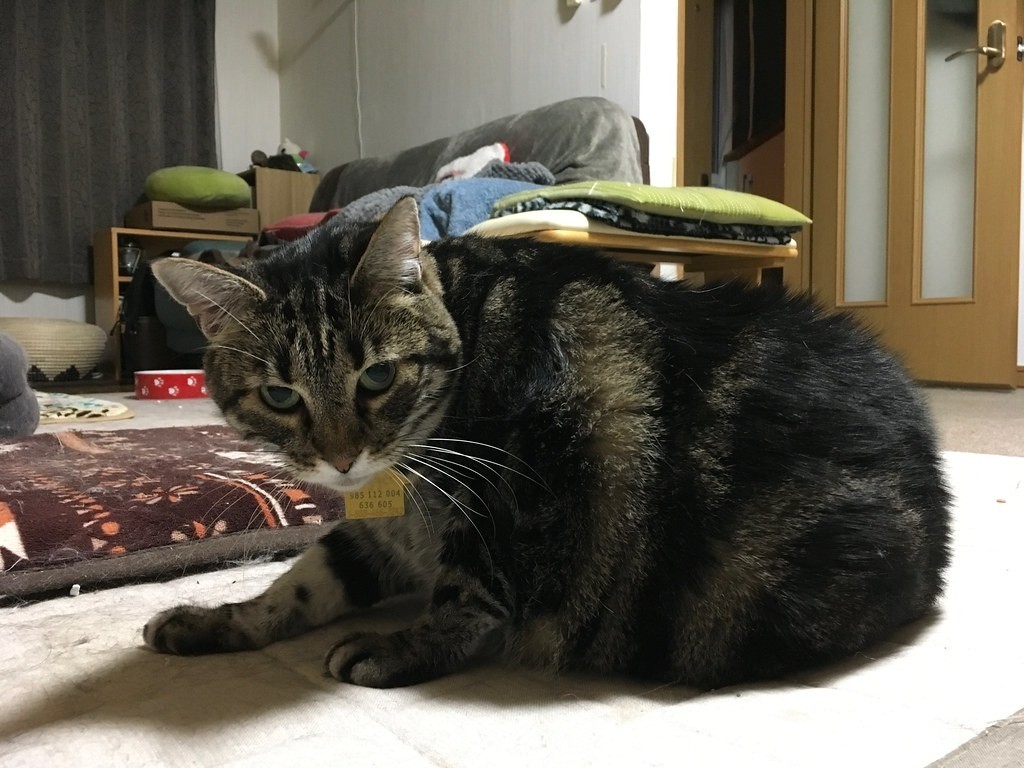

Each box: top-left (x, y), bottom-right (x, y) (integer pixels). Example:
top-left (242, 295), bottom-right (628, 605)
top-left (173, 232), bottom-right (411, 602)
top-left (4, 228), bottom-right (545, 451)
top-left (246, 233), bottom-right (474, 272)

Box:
top-left (144, 165), bottom-right (252, 207)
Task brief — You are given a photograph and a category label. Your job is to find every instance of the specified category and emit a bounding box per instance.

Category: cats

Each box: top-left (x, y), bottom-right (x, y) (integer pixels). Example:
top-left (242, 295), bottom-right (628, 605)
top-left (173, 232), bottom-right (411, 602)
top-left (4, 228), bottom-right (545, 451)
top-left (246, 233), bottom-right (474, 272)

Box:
top-left (139, 193), bottom-right (956, 691)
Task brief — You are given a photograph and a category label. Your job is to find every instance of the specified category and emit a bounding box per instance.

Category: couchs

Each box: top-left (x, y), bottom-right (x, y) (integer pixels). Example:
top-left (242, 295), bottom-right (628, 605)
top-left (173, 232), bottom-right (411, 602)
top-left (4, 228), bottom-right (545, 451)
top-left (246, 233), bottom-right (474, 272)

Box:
top-left (116, 96), bottom-right (649, 378)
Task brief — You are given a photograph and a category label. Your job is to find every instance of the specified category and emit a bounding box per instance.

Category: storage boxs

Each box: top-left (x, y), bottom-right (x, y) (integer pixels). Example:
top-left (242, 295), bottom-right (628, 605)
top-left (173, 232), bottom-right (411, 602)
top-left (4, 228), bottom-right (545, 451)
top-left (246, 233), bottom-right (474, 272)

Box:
top-left (133, 369), bottom-right (212, 399)
top-left (123, 200), bottom-right (261, 235)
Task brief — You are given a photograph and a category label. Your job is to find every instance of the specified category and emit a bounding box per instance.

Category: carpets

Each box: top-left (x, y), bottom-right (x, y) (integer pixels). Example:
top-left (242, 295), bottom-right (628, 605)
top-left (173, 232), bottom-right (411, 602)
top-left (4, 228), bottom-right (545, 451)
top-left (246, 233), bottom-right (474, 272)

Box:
top-left (1, 447), bottom-right (1024, 768)
top-left (34, 390), bottom-right (136, 424)
top-left (0, 423), bottom-right (346, 606)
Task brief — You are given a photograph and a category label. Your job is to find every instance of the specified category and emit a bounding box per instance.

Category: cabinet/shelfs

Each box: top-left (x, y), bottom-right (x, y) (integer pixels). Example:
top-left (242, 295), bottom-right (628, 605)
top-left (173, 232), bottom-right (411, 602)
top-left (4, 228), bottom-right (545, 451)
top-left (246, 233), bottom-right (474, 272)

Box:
top-left (92, 227), bottom-right (254, 385)
top-left (236, 166), bottom-right (322, 235)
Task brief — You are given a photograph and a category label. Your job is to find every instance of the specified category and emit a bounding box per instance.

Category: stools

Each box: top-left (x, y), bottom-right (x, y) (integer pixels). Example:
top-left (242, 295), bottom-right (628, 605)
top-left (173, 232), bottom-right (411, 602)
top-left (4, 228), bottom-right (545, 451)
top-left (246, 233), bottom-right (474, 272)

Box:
top-left (490, 229), bottom-right (799, 289)
